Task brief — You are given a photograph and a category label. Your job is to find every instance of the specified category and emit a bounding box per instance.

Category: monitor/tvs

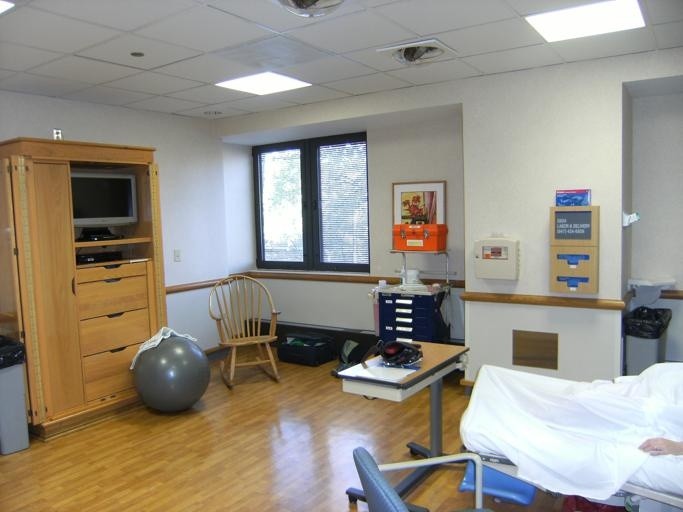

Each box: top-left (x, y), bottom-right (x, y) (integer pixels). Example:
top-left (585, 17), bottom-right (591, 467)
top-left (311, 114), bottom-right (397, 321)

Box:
top-left (71, 172), bottom-right (138, 242)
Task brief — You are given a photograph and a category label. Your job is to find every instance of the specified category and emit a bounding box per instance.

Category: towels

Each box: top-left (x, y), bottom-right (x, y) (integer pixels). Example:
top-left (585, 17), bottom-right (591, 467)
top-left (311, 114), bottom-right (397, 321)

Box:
top-left (128, 325), bottom-right (198, 371)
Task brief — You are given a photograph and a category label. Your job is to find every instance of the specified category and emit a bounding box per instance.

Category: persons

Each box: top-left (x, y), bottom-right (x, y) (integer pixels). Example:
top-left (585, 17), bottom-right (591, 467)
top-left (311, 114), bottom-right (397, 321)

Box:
top-left (639, 437), bottom-right (683, 456)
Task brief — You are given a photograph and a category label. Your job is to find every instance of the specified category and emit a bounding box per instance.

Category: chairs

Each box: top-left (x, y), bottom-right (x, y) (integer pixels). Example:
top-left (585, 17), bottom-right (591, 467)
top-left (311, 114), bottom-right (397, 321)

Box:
top-left (208, 273), bottom-right (283, 390)
top-left (350, 443), bottom-right (494, 511)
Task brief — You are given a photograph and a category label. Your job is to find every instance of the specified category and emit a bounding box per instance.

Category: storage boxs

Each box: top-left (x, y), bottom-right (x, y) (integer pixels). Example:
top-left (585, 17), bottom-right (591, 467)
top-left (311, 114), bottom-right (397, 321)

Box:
top-left (391, 223), bottom-right (448, 251)
top-left (276, 329), bottom-right (337, 366)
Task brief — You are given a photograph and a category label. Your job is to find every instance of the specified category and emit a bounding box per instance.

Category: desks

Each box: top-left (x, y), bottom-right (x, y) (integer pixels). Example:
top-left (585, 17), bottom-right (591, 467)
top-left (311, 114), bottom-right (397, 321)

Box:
top-left (333, 337), bottom-right (471, 512)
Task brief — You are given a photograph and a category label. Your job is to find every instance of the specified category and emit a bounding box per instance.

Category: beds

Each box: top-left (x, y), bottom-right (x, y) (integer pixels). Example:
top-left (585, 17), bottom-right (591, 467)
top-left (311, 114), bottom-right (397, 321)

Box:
top-left (458, 361), bottom-right (683, 511)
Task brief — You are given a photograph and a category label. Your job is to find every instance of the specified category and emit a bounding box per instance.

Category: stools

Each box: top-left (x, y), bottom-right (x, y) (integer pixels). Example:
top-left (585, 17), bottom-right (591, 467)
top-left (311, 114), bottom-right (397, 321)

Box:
top-left (458, 459), bottom-right (539, 507)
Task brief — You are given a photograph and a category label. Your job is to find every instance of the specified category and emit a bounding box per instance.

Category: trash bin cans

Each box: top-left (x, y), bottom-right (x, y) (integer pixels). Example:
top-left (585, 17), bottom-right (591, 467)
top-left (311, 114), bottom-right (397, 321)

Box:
top-left (0, 334), bottom-right (30, 456)
top-left (622, 305), bottom-right (672, 376)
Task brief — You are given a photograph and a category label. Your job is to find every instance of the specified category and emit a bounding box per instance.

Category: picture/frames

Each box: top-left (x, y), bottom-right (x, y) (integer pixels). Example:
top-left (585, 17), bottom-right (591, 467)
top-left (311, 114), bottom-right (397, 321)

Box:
top-left (390, 181), bottom-right (447, 224)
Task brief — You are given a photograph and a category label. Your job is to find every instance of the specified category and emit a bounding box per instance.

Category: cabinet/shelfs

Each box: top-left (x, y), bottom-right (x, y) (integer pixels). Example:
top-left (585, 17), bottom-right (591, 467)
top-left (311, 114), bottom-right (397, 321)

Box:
top-left (373, 250), bottom-right (450, 345)
top-left (0, 138), bottom-right (168, 440)
